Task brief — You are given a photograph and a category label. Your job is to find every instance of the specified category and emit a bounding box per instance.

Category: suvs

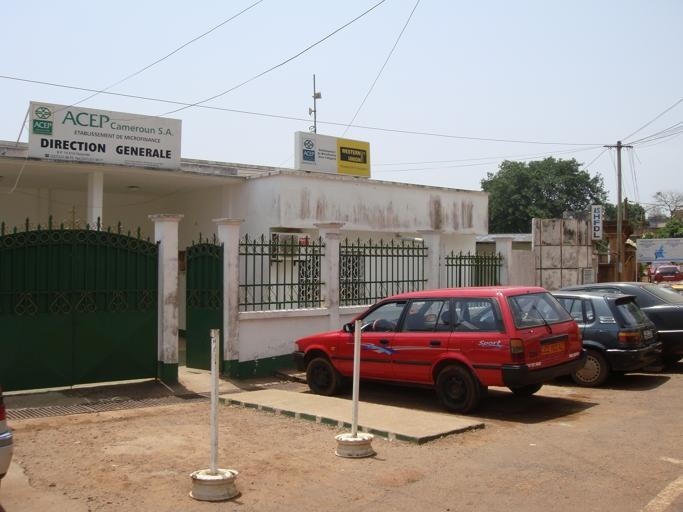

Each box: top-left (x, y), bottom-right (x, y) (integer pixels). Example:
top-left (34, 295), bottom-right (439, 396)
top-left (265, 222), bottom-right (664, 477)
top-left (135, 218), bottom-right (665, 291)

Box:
top-left (293, 286), bottom-right (586, 414)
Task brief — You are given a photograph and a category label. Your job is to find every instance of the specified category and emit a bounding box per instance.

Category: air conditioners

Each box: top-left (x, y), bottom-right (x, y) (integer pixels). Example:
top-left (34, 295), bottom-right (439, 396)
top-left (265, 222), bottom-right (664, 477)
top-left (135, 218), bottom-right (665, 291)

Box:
top-left (269, 232), bottom-right (310, 263)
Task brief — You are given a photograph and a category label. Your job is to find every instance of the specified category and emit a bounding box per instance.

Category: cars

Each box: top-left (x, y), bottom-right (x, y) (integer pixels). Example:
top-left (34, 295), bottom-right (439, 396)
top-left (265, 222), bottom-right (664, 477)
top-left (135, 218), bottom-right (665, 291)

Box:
top-left (642, 260), bottom-right (682, 284)
top-left (0, 385), bottom-right (14, 479)
top-left (516, 280), bottom-right (683, 373)
top-left (469, 290), bottom-right (662, 388)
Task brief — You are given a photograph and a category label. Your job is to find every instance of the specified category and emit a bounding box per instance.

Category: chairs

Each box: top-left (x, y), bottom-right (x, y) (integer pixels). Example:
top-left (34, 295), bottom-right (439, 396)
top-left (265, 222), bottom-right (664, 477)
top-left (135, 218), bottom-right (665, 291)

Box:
top-left (439, 310), bottom-right (457, 328)
top-left (546, 299), bottom-right (566, 320)
top-left (406, 313), bottom-right (424, 328)
